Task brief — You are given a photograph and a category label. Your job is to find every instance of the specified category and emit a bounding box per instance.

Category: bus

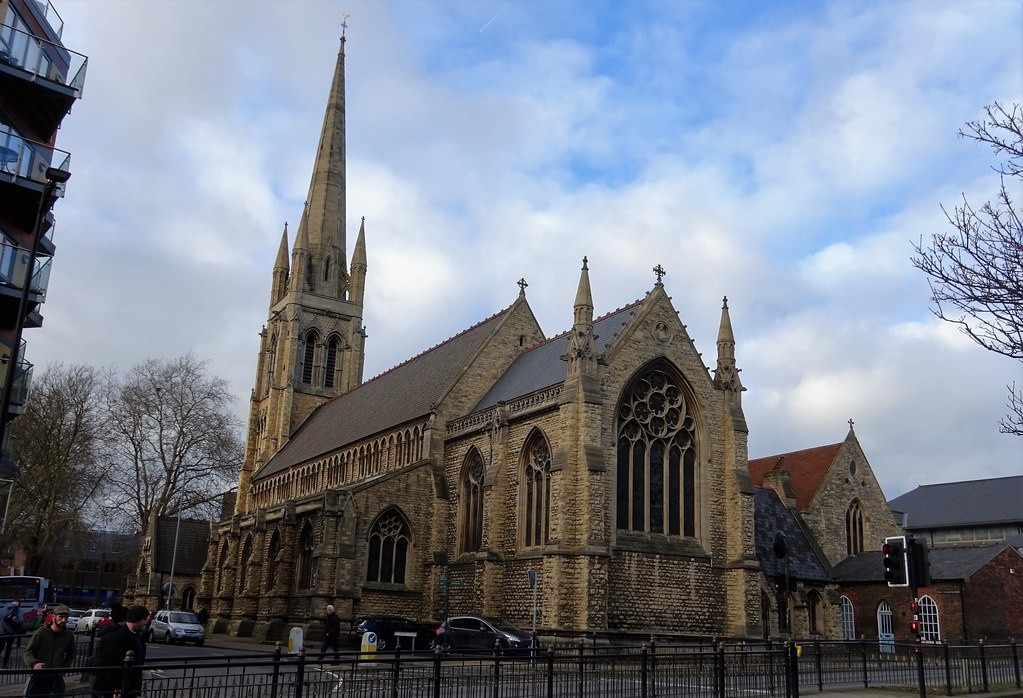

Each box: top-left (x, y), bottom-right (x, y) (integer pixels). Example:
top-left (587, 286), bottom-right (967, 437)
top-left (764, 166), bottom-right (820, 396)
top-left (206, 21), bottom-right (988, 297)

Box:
top-left (0, 575), bottom-right (49, 629)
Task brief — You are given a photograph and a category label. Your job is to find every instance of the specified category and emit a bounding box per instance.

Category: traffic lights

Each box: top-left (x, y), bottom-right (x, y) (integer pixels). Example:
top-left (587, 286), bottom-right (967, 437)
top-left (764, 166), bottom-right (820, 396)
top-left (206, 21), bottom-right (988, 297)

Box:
top-left (905, 537), bottom-right (931, 588)
top-left (882, 535), bottom-right (909, 588)
top-left (909, 622), bottom-right (919, 634)
top-left (910, 601), bottom-right (916, 611)
top-left (913, 645), bottom-right (921, 655)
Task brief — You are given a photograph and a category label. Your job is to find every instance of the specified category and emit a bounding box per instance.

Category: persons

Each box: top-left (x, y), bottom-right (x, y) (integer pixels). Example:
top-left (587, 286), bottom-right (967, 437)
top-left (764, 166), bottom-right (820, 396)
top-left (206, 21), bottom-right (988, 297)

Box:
top-left (22, 603), bottom-right (76, 698)
top-left (105, 605), bottom-right (153, 696)
top-left (90, 604), bottom-right (129, 698)
top-left (315, 603), bottom-right (340, 666)
top-left (0, 597), bottom-right (26, 669)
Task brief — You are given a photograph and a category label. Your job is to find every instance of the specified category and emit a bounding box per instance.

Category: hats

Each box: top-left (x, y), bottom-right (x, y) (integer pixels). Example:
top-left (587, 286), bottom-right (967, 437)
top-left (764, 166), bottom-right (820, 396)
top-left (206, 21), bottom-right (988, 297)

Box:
top-left (110, 604), bottom-right (130, 622)
top-left (127, 605), bottom-right (150, 622)
top-left (53, 604), bottom-right (70, 615)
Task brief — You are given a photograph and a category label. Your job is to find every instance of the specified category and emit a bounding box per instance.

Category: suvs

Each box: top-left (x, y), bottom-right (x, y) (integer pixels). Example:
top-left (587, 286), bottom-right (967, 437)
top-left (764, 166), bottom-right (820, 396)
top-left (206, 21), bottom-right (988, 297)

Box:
top-left (75, 608), bottom-right (112, 636)
top-left (148, 610), bottom-right (205, 648)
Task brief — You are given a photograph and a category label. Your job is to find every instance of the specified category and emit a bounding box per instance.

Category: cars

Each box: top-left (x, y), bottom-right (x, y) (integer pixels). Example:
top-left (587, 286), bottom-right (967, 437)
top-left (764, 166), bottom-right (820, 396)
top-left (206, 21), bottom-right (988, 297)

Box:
top-left (66, 610), bottom-right (85, 630)
top-left (433, 616), bottom-right (539, 660)
top-left (351, 614), bottom-right (433, 650)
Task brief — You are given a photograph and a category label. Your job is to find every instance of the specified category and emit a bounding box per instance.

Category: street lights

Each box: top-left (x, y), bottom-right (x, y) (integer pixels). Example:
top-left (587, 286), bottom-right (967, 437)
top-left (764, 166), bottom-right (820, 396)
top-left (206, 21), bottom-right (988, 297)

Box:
top-left (141, 458), bottom-right (188, 609)
